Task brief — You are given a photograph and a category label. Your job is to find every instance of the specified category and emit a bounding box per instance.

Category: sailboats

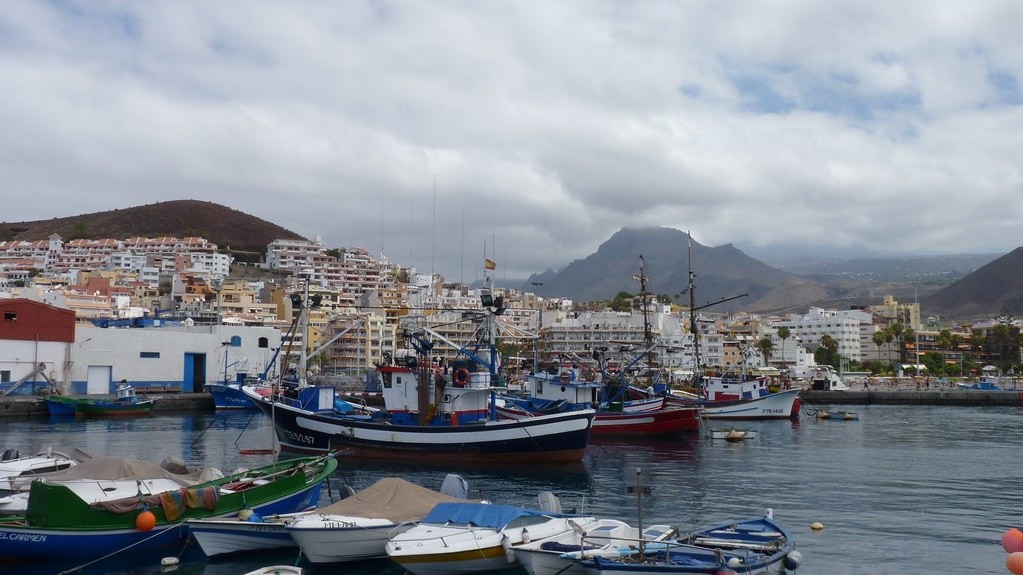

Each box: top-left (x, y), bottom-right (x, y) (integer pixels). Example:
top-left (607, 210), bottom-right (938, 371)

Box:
top-left (664, 228), bottom-right (805, 418)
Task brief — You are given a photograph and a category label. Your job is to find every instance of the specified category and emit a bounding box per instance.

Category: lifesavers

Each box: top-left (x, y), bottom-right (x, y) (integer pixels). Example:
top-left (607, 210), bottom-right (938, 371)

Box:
top-left (559, 371), bottom-right (571, 385)
top-left (817, 372), bottom-right (822, 378)
top-left (585, 369), bottom-right (597, 382)
top-left (454, 367), bottom-right (471, 385)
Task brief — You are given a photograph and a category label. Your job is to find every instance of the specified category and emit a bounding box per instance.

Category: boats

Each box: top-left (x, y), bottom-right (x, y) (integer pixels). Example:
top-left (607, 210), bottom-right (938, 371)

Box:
top-left (1, 447), bottom-right (339, 575)
top-left (710, 428), bottom-right (758, 439)
top-left (486, 394), bottom-right (702, 438)
top-left (600, 398), bottom-right (665, 412)
top-left (816, 409), bottom-right (859, 421)
top-left (184, 491), bottom-right (316, 562)
top-left (204, 342), bottom-right (286, 410)
top-left (37, 382), bottom-right (161, 417)
top-left (562, 511), bottom-right (804, 574)
top-left (956, 375), bottom-right (1016, 391)
top-left (509, 517), bottom-right (680, 575)
top-left (286, 478), bottom-right (491, 573)
top-left (383, 500), bottom-right (595, 575)
top-left (806, 365), bottom-right (850, 391)
top-left (239, 274), bottom-right (598, 465)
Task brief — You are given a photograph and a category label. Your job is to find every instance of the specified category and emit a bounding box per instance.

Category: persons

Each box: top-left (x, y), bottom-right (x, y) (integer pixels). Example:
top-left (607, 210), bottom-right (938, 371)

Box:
top-left (647, 385), bottom-right (654, 398)
top-left (784, 377), bottom-right (792, 390)
top-left (864, 377), bottom-right (868, 389)
top-left (824, 376), bottom-right (829, 391)
top-left (1013, 378), bottom-right (1016, 389)
top-left (810, 376), bottom-right (814, 390)
top-left (926, 380), bottom-right (929, 389)
top-left (916, 380), bottom-right (920, 388)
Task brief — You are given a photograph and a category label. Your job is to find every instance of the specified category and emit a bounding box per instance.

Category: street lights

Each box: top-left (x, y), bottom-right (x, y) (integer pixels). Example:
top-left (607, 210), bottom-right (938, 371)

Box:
top-left (911, 280), bottom-right (921, 376)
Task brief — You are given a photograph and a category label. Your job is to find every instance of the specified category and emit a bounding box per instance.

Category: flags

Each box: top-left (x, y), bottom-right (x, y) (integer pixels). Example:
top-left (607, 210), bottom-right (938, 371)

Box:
top-left (485, 259), bottom-right (496, 270)
